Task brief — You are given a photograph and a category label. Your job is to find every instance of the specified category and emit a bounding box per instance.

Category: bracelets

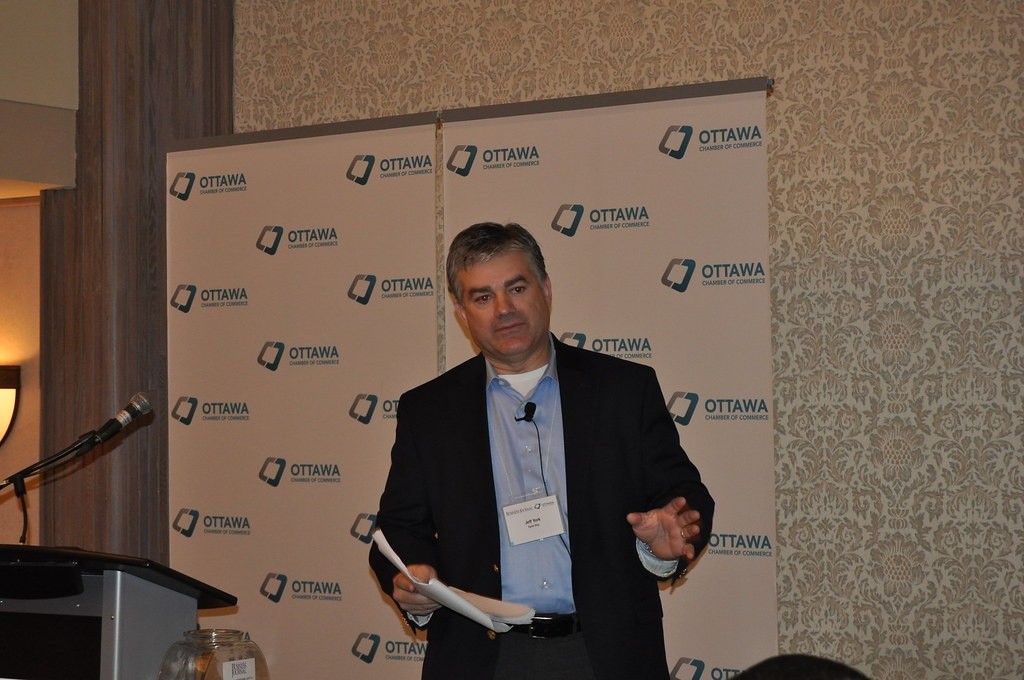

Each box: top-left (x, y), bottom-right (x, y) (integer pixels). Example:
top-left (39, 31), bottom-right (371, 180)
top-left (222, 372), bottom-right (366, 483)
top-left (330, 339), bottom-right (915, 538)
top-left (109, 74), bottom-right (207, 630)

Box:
top-left (642, 542), bottom-right (653, 554)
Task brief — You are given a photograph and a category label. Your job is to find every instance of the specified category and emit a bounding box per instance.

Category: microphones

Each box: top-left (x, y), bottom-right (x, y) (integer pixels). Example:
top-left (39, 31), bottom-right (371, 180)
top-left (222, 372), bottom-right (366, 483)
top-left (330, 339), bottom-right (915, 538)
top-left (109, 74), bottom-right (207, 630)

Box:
top-left (523, 402), bottom-right (538, 421)
top-left (76, 391), bottom-right (152, 457)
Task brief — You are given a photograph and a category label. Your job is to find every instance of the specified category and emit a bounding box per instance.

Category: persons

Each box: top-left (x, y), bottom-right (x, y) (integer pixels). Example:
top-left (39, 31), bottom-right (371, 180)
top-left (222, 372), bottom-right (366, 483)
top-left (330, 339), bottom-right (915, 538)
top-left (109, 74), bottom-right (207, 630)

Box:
top-left (369, 221), bottom-right (712, 680)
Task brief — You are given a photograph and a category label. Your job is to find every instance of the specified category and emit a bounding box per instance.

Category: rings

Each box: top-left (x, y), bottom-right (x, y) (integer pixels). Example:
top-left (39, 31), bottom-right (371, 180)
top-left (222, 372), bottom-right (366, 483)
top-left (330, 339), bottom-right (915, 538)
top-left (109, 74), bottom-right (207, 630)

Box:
top-left (681, 529), bottom-right (685, 538)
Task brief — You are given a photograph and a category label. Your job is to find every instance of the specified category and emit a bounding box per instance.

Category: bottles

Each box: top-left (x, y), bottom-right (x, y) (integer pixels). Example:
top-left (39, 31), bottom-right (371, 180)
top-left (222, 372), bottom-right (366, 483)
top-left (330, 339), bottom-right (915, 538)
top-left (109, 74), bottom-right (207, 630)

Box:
top-left (157, 630), bottom-right (270, 680)
